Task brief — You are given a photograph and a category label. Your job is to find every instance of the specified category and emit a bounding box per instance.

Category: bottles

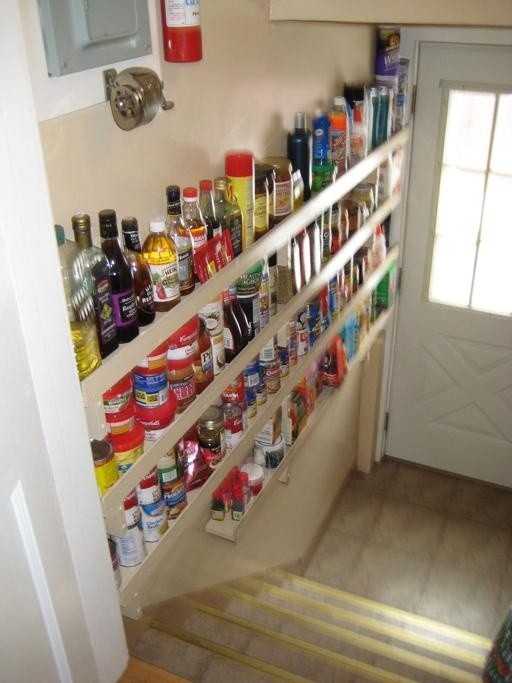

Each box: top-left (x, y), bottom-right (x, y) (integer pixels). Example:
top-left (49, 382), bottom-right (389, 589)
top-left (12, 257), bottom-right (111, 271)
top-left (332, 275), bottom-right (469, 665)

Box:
top-left (212, 462), bottom-right (263, 522)
top-left (372, 225), bottom-right (388, 261)
top-left (54, 82), bottom-right (398, 379)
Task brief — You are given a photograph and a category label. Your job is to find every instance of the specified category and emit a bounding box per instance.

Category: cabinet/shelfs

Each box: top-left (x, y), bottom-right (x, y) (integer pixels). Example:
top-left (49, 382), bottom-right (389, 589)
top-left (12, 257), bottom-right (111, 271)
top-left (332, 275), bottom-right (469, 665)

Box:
top-left (81, 126), bottom-right (414, 609)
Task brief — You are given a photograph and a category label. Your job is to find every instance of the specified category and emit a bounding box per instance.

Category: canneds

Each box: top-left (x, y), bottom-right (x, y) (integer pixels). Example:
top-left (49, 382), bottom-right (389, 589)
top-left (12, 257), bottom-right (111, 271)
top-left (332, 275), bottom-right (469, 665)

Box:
top-left (219, 402), bottom-right (243, 449)
top-left (261, 158), bottom-right (293, 225)
top-left (198, 428), bottom-right (221, 466)
top-left (198, 405), bottom-right (226, 458)
